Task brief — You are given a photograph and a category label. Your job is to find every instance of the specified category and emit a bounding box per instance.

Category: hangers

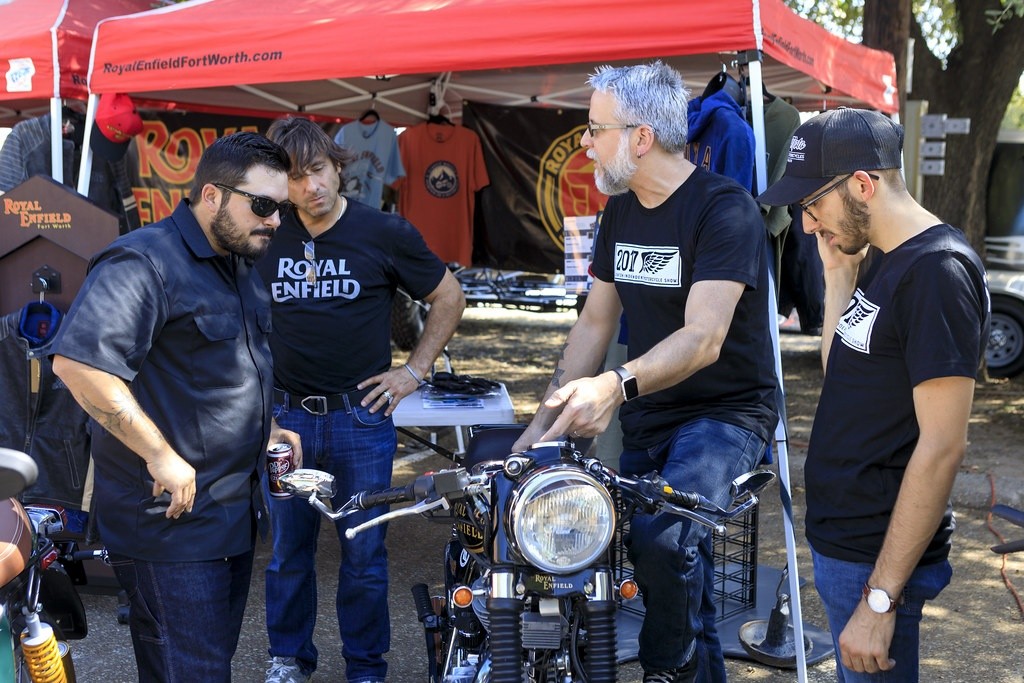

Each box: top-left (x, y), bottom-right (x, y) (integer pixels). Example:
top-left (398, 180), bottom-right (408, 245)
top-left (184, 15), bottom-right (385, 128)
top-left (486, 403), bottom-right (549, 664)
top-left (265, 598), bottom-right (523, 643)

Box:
top-left (736, 62), bottom-right (775, 100)
top-left (61, 99), bottom-right (85, 128)
top-left (0, 277), bottom-right (66, 328)
top-left (359, 103), bottom-right (380, 124)
top-left (426, 113), bottom-right (455, 126)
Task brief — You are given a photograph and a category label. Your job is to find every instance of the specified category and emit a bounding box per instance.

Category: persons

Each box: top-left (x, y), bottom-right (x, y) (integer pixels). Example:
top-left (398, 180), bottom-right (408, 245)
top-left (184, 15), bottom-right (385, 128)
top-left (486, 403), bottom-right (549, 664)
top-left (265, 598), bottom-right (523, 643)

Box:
top-left (510, 60), bottom-right (781, 683)
top-left (753, 106), bottom-right (990, 683)
top-left (46, 132), bottom-right (306, 683)
top-left (255, 118), bottom-right (465, 683)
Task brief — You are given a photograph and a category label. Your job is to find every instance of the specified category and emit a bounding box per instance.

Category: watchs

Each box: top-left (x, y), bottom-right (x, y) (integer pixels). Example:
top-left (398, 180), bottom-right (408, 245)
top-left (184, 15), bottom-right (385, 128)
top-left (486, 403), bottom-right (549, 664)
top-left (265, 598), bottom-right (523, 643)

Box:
top-left (862, 581), bottom-right (896, 613)
top-left (611, 366), bottom-right (639, 401)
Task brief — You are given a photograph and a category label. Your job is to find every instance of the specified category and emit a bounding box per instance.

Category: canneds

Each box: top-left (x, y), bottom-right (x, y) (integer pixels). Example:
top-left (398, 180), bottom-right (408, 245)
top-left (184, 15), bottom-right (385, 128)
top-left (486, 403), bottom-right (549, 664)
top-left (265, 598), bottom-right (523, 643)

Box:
top-left (267, 443), bottom-right (295, 498)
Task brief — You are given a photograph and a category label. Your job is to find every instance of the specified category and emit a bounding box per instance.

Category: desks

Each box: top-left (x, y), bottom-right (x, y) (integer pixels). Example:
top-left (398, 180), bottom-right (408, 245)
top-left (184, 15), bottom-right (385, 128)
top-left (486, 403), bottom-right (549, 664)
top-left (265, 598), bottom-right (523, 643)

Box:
top-left (389, 381), bottom-right (515, 457)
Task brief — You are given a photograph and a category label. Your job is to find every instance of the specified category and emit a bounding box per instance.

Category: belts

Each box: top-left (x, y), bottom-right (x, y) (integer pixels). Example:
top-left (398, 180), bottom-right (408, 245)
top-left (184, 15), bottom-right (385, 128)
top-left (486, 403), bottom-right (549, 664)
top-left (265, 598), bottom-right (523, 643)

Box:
top-left (273, 384), bottom-right (383, 416)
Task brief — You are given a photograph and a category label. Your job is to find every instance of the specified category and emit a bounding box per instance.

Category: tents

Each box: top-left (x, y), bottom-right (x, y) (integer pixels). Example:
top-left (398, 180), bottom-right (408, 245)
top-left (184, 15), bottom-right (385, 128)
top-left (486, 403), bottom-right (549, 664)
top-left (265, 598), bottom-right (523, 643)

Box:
top-left (0, 0), bottom-right (899, 683)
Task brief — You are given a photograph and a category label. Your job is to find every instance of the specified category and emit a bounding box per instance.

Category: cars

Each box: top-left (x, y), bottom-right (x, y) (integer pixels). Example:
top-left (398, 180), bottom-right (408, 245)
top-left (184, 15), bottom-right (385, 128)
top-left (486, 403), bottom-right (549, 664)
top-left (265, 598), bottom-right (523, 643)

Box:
top-left (981, 267), bottom-right (1024, 377)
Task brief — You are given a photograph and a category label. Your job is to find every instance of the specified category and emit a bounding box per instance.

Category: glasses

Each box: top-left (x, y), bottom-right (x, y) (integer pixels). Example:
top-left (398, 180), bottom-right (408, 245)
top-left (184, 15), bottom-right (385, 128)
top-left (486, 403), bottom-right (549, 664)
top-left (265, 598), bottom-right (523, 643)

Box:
top-left (301, 238), bottom-right (318, 289)
top-left (798, 170), bottom-right (880, 222)
top-left (588, 120), bottom-right (656, 138)
top-left (201, 182), bottom-right (293, 225)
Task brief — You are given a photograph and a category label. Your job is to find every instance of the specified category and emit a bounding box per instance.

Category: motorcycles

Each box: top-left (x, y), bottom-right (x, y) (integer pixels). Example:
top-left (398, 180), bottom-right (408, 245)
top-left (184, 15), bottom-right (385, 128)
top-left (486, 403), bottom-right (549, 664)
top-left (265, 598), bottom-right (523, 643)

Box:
top-left (280, 425), bottom-right (778, 683)
top-left (0, 498), bottom-right (88, 682)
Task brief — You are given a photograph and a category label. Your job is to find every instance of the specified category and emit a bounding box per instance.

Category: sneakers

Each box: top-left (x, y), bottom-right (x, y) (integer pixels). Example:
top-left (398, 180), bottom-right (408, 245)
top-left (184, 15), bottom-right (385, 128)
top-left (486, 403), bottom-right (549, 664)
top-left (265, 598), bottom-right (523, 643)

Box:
top-left (264, 656), bottom-right (313, 683)
top-left (643, 650), bottom-right (699, 683)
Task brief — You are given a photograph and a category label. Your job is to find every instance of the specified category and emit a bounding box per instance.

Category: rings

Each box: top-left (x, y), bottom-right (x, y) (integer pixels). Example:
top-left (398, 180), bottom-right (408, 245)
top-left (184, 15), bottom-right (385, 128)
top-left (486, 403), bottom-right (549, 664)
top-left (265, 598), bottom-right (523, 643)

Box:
top-left (383, 391), bottom-right (392, 399)
top-left (569, 431), bottom-right (580, 439)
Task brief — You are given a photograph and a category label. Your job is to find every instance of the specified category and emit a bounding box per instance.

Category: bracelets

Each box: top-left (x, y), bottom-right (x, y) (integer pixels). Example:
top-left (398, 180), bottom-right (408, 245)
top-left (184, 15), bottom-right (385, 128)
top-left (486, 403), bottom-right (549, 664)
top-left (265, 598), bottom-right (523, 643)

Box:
top-left (405, 364), bottom-right (422, 384)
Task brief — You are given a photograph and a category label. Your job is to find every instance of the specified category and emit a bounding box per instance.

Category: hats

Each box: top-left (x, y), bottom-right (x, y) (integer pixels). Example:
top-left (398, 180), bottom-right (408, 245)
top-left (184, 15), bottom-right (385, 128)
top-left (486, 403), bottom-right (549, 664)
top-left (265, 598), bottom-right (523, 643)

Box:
top-left (89, 93), bottom-right (144, 162)
top-left (755, 106), bottom-right (902, 205)
